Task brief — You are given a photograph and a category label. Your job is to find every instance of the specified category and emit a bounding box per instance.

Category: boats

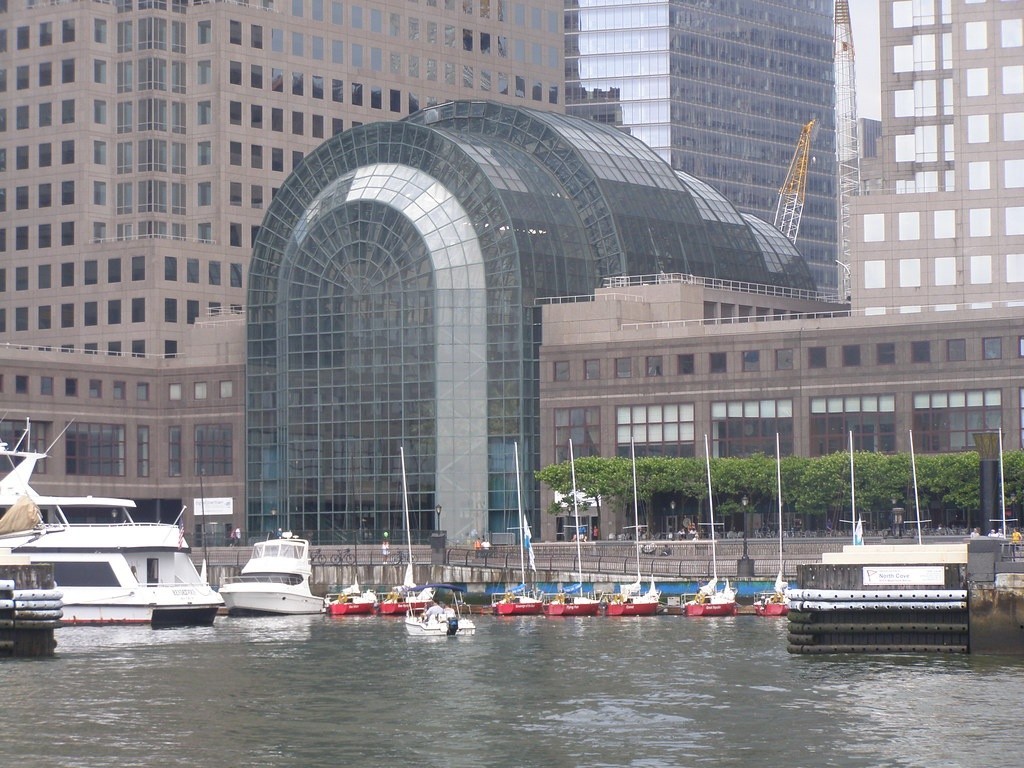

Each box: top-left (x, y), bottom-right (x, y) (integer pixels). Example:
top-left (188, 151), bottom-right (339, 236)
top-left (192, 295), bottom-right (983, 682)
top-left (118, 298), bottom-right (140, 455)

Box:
top-left (405, 584), bottom-right (478, 638)
top-left (1, 417), bottom-right (227, 629)
top-left (217, 531), bottom-right (325, 617)
top-left (323, 583), bottom-right (376, 617)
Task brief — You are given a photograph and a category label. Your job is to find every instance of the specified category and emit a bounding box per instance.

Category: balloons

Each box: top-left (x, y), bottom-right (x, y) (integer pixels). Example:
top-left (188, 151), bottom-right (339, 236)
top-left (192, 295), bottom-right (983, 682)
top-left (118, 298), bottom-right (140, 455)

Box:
top-left (384, 532), bottom-right (388, 540)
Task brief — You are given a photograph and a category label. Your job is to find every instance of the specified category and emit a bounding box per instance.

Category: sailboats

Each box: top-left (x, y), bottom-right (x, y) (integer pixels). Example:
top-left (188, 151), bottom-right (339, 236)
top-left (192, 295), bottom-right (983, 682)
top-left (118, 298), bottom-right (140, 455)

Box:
top-left (380, 446), bottom-right (435, 614)
top-left (680, 434), bottom-right (738, 617)
top-left (489, 441), bottom-right (545, 617)
top-left (606, 437), bottom-right (659, 616)
top-left (753, 432), bottom-right (793, 616)
top-left (547, 437), bottom-right (601, 616)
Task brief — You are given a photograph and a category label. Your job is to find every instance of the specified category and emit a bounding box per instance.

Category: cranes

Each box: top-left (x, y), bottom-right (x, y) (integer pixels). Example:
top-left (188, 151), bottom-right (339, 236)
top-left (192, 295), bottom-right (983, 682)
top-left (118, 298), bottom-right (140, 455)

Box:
top-left (767, 118), bottom-right (816, 248)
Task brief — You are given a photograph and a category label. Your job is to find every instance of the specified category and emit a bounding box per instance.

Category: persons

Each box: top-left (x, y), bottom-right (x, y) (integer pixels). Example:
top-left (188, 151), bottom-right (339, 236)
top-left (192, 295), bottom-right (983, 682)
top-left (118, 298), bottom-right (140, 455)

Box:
top-left (229, 526), bottom-right (241, 547)
top-left (1011, 527), bottom-right (1022, 562)
top-left (382, 540), bottom-right (390, 562)
top-left (695, 589), bottom-right (705, 604)
top-left (988, 529), bottom-right (999, 538)
top-left (869, 520), bottom-right (965, 537)
top-left (572, 525), bottom-right (697, 542)
top-left (277, 528), bottom-right (283, 539)
top-left (421, 598), bottom-right (456, 622)
top-left (996, 529), bottom-right (1004, 538)
top-left (505, 589), bottom-right (514, 602)
top-left (473, 538), bottom-right (491, 559)
top-left (759, 518), bottom-right (833, 537)
top-left (970, 527), bottom-right (980, 539)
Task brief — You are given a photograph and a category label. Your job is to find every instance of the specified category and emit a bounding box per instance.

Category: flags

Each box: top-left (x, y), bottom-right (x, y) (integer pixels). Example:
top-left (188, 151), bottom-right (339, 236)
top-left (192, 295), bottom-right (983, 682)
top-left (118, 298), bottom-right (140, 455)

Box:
top-left (854, 519), bottom-right (865, 545)
top-left (178, 516), bottom-right (185, 551)
top-left (522, 515), bottom-right (537, 572)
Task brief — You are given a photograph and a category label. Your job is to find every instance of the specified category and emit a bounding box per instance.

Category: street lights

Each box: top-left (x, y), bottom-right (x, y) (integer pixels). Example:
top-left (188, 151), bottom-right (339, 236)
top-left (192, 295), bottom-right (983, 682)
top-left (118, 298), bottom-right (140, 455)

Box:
top-left (435, 503), bottom-right (442, 535)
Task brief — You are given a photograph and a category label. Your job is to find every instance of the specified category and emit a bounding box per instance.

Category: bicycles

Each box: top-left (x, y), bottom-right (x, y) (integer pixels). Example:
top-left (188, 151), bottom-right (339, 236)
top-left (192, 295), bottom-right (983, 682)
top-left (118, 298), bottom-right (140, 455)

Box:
top-left (308, 548), bottom-right (327, 563)
top-left (388, 547), bottom-right (419, 565)
top-left (330, 548), bottom-right (356, 564)
